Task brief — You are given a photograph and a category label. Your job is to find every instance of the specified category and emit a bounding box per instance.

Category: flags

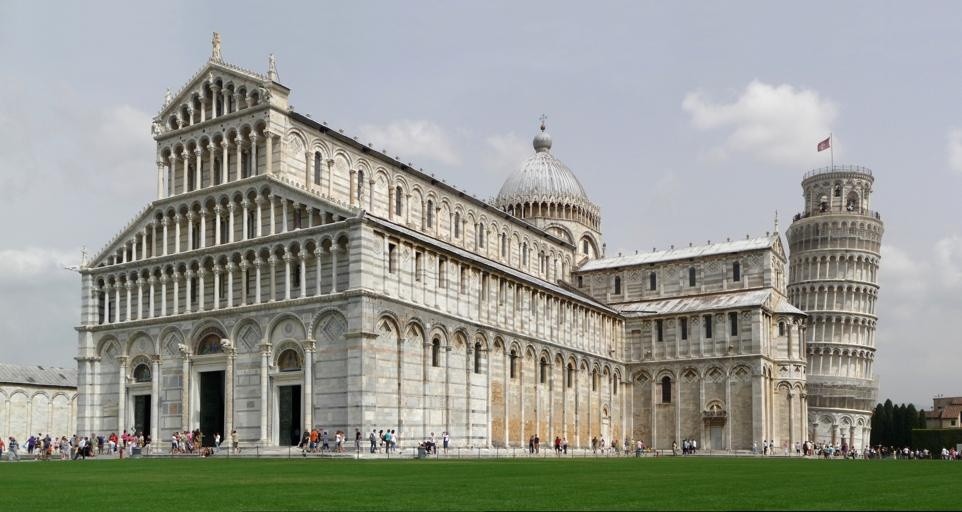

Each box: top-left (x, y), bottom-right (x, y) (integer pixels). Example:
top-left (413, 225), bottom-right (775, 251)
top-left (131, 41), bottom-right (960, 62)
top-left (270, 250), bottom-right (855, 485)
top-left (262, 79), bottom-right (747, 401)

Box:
top-left (816, 136), bottom-right (830, 151)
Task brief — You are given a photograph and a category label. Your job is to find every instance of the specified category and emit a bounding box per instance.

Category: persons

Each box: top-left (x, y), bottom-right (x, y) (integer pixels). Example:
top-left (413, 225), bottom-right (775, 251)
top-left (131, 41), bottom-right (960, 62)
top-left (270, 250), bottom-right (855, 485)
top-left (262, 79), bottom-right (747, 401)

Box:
top-left (0, 429), bottom-right (240, 460)
top-left (211, 31), bottom-right (222, 58)
top-left (269, 52), bottom-right (276, 70)
top-left (750, 436), bottom-right (962, 461)
top-left (522, 434), bottom-right (697, 454)
top-left (164, 87), bottom-right (171, 105)
top-left (298, 427), bottom-right (450, 458)
